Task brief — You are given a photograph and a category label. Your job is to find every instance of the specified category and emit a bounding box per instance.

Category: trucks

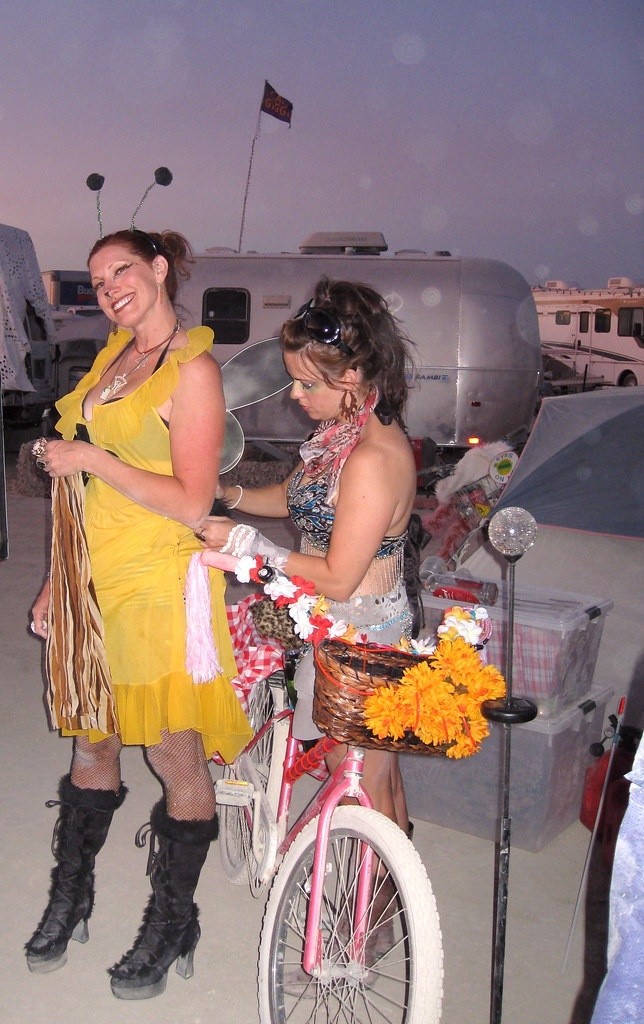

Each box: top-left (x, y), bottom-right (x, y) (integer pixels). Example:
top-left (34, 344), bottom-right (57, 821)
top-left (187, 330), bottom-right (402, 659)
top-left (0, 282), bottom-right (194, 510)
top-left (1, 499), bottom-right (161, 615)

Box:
top-left (40, 232), bottom-right (543, 484)
top-left (0, 222), bottom-right (57, 428)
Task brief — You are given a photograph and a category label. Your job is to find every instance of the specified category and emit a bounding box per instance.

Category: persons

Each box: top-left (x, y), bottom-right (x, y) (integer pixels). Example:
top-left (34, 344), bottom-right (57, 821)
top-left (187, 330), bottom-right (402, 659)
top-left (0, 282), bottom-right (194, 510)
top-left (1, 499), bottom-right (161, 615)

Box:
top-left (25, 228), bottom-right (224, 1000)
top-left (193, 280), bottom-right (416, 989)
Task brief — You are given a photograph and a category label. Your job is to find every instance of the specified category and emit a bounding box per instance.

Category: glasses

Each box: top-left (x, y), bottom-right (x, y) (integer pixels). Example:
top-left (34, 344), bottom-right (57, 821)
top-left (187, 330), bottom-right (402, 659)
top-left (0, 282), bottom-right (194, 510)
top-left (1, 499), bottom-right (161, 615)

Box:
top-left (292, 298), bottom-right (357, 357)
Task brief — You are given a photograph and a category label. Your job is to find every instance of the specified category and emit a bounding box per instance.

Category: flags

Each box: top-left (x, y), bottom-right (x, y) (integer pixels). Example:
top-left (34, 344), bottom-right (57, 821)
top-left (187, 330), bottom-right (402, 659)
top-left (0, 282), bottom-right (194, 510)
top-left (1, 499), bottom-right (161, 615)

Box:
top-left (261, 81), bottom-right (294, 124)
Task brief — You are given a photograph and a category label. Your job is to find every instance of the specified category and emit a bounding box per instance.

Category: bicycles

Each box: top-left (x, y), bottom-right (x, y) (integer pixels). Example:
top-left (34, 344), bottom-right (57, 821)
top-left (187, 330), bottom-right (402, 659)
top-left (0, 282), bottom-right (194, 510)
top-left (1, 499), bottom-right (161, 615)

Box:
top-left (416, 424), bottom-right (527, 534)
top-left (211, 594), bottom-right (469, 1024)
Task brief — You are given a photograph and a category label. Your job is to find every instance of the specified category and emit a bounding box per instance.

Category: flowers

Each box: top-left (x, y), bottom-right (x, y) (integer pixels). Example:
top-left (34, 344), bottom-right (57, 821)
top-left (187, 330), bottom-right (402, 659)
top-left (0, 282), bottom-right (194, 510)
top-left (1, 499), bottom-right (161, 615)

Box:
top-left (365, 625), bottom-right (507, 760)
top-left (234, 548), bottom-right (484, 657)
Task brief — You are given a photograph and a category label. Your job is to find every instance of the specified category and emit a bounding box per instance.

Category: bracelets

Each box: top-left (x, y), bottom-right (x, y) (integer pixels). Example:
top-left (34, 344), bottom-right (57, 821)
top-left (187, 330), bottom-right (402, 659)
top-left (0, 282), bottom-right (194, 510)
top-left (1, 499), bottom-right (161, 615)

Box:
top-left (227, 485), bottom-right (243, 510)
top-left (47, 572), bottom-right (50, 577)
top-left (219, 524), bottom-right (258, 557)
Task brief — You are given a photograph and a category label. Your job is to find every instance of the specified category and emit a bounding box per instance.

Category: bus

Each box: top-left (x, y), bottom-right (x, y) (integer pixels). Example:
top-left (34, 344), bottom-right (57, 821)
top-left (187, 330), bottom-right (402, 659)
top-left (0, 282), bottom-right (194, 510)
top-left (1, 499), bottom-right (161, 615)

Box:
top-left (529, 277), bottom-right (644, 387)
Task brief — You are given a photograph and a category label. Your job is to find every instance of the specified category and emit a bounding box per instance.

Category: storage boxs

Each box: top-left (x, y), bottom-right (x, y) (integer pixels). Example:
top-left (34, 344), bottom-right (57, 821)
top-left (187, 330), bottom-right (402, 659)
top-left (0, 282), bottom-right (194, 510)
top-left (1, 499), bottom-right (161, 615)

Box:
top-left (395, 569), bottom-right (618, 853)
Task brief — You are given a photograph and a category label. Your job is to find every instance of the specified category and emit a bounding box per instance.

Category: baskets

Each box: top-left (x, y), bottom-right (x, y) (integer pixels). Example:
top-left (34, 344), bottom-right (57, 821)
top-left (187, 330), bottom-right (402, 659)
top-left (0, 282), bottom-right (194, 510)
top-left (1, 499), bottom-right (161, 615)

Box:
top-left (312, 636), bottom-right (465, 760)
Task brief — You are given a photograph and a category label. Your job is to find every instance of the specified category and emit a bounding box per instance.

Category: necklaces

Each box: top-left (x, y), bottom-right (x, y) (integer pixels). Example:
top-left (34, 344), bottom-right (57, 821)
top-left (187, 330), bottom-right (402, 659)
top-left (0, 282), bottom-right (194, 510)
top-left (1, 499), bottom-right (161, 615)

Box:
top-left (100, 317), bottom-right (182, 400)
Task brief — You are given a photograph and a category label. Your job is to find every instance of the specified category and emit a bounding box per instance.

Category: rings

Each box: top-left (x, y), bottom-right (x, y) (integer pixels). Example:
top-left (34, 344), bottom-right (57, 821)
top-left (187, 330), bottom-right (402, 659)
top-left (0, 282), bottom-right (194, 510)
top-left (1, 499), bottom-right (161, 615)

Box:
top-left (31, 620), bottom-right (47, 634)
top-left (35, 458), bottom-right (46, 469)
top-left (195, 529), bottom-right (207, 541)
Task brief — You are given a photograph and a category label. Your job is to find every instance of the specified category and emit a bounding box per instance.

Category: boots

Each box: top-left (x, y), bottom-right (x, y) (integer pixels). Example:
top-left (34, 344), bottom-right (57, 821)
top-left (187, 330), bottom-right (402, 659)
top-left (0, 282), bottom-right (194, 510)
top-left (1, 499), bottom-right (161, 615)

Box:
top-left (106, 795), bottom-right (220, 999)
top-left (341, 821), bottom-right (414, 960)
top-left (281, 875), bottom-right (394, 998)
top-left (22, 772), bottom-right (129, 974)
